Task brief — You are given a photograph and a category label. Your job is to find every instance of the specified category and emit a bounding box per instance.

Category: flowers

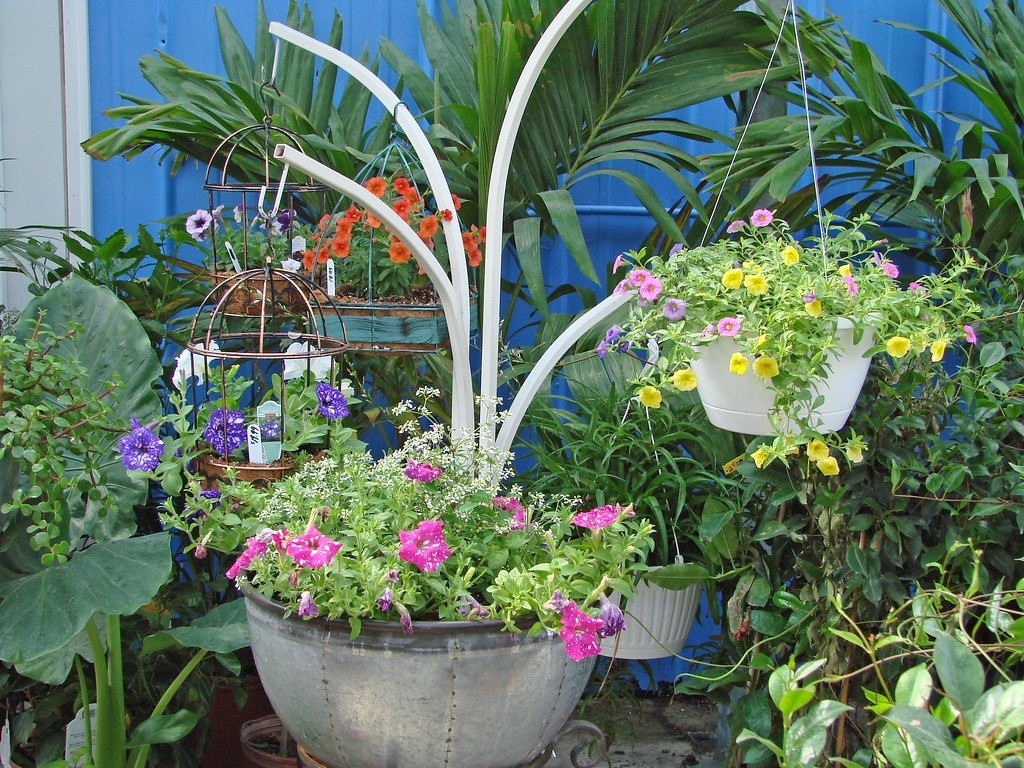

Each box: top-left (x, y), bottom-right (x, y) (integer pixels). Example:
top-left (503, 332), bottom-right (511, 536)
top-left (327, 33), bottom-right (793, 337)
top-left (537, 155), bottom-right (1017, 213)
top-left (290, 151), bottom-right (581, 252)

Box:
top-left (596, 208), bottom-right (978, 478)
top-left (184, 205), bottom-right (315, 272)
top-left (116, 339), bottom-right (361, 518)
top-left (156, 387), bottom-right (661, 665)
top-left (301, 176), bottom-right (487, 300)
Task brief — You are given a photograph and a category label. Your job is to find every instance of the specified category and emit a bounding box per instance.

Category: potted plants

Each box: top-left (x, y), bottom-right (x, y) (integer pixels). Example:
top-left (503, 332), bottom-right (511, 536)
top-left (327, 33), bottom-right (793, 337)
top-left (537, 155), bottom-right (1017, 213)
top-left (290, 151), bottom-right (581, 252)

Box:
top-left (129, 488), bottom-right (277, 767)
top-left (530, 388), bottom-right (718, 660)
top-left (239, 714), bottom-right (298, 768)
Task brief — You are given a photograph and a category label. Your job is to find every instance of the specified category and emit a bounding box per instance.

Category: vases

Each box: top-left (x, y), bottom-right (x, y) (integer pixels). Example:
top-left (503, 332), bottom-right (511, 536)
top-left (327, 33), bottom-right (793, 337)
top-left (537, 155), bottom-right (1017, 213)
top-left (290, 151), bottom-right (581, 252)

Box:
top-left (205, 271), bottom-right (317, 316)
top-left (684, 317), bottom-right (878, 436)
top-left (237, 580), bottom-right (596, 768)
top-left (307, 285), bottom-right (478, 357)
top-left (203, 455), bottom-right (325, 512)
